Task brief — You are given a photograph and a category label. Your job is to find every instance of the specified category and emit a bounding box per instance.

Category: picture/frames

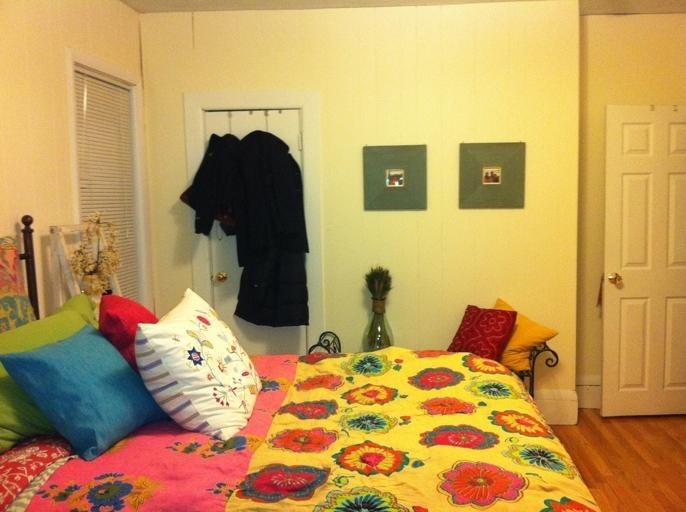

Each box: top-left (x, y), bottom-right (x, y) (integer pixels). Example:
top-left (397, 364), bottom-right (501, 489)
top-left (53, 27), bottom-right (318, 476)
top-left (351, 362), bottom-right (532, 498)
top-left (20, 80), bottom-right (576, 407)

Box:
top-left (459, 143), bottom-right (526, 209)
top-left (50, 223), bottom-right (123, 321)
top-left (363, 145), bottom-right (427, 211)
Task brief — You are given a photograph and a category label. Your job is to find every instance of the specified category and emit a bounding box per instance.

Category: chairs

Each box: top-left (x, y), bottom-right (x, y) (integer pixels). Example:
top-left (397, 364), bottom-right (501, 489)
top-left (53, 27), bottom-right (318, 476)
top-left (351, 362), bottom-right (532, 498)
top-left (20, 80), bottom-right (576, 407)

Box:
top-left (309, 331), bottom-right (342, 355)
top-left (510, 342), bottom-right (559, 399)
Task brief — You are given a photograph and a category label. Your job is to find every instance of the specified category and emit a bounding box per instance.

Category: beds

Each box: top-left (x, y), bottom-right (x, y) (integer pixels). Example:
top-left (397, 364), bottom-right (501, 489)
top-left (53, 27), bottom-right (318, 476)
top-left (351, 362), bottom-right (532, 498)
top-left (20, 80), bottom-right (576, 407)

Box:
top-left (0, 215), bottom-right (600, 512)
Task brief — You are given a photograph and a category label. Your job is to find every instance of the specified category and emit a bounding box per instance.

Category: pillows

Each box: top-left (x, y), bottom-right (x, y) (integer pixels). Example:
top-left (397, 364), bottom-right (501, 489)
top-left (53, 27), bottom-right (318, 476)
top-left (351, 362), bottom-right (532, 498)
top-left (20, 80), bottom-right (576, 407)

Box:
top-left (135, 288), bottom-right (262, 442)
top-left (99, 295), bottom-right (160, 372)
top-left (447, 305), bottom-right (517, 363)
top-left (0, 295), bottom-right (99, 456)
top-left (0, 325), bottom-right (172, 461)
top-left (492, 299), bottom-right (558, 371)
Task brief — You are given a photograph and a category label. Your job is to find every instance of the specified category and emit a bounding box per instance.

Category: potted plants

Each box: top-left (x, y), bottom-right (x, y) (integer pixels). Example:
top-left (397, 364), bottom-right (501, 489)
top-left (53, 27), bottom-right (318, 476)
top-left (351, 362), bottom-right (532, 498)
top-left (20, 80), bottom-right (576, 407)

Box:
top-left (363, 266), bottom-right (394, 352)
